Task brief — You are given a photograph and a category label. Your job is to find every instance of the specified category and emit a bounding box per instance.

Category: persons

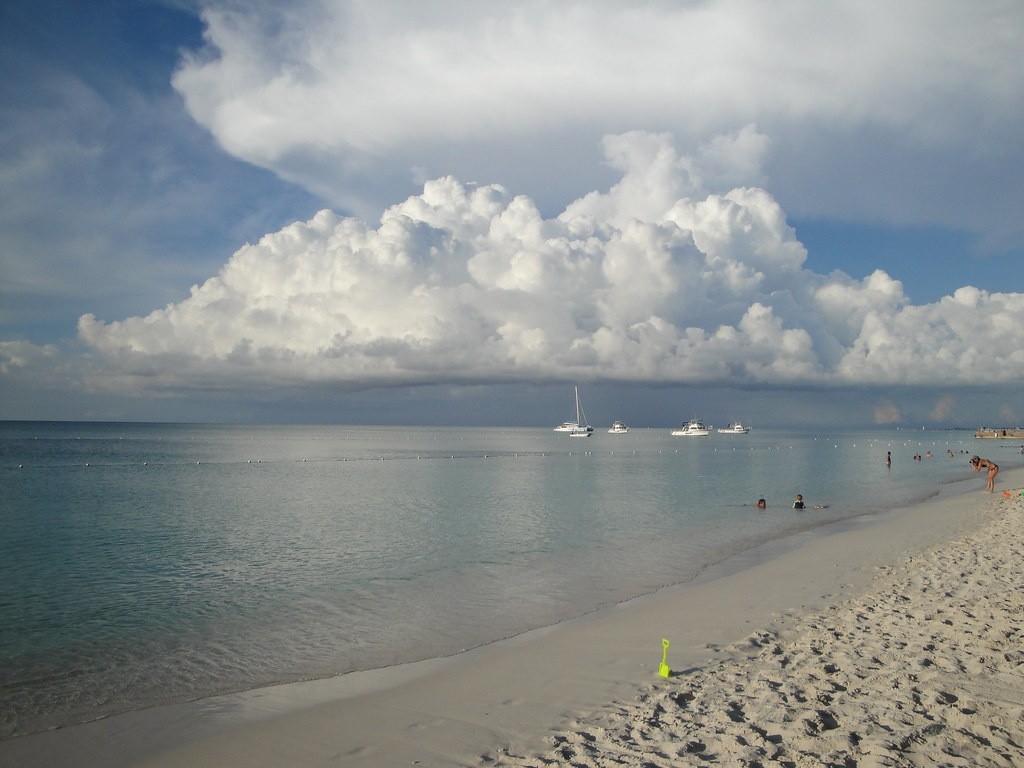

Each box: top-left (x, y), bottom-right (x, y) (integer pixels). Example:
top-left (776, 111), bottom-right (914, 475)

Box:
top-left (970, 455), bottom-right (999, 493)
top-left (886, 452), bottom-right (891, 466)
top-left (792, 494), bottom-right (805, 509)
top-left (758, 499), bottom-right (766, 509)
top-left (913, 444), bottom-right (1024, 461)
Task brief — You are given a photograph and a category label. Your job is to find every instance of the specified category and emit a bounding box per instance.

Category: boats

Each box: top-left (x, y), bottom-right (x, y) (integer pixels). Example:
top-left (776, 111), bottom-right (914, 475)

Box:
top-left (671, 417), bottom-right (710, 436)
top-left (718, 422), bottom-right (752, 434)
top-left (607, 421), bottom-right (629, 433)
top-left (570, 433), bottom-right (593, 437)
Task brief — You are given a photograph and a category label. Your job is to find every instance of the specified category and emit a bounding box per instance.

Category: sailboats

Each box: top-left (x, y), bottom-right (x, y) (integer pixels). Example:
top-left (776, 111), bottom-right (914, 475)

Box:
top-left (552, 386), bottom-right (594, 432)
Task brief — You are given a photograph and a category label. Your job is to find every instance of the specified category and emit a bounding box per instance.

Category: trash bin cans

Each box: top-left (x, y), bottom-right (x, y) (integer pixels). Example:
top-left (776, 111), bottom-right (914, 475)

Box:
top-left (1003, 430), bottom-right (1006, 436)
top-left (995, 433), bottom-right (998, 437)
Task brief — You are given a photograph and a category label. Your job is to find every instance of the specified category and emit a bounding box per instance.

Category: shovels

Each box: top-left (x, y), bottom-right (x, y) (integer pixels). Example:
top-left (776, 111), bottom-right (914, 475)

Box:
top-left (659, 639), bottom-right (671, 677)
